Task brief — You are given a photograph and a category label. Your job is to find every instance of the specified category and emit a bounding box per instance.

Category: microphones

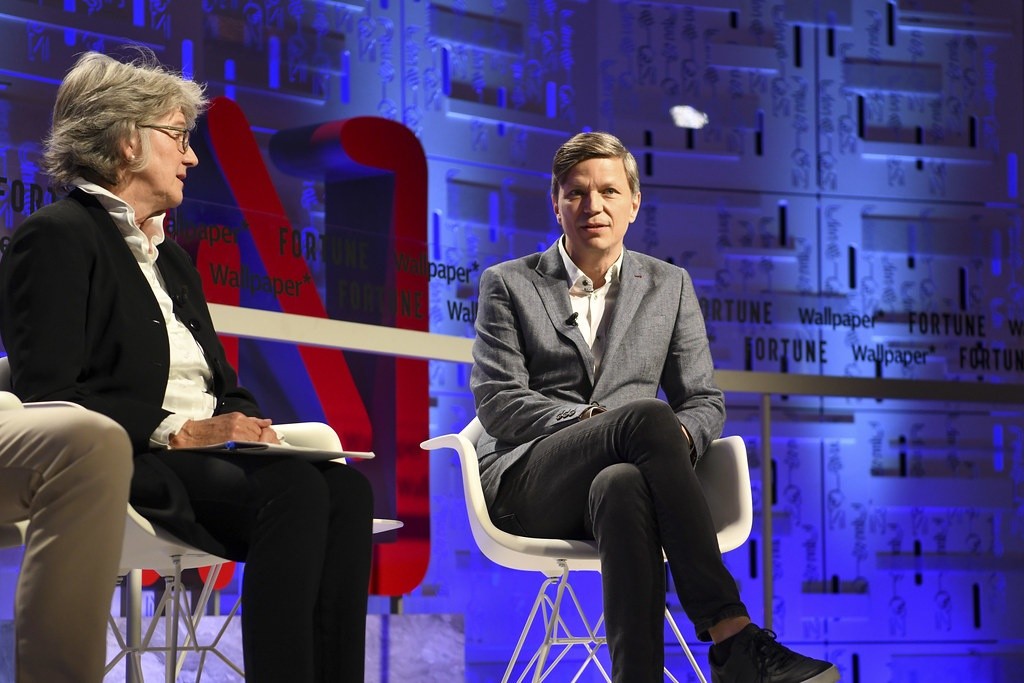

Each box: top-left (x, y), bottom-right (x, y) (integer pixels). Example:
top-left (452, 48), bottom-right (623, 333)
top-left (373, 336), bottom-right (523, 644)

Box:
top-left (565, 311), bottom-right (579, 326)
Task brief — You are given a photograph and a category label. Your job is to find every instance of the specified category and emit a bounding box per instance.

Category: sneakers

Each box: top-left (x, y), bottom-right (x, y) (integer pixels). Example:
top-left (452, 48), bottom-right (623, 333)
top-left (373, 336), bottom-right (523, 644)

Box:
top-left (707, 624), bottom-right (841, 683)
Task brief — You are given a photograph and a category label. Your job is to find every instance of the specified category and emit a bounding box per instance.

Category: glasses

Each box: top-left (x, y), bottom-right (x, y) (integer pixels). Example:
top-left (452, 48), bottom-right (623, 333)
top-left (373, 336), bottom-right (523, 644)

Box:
top-left (137, 123), bottom-right (191, 151)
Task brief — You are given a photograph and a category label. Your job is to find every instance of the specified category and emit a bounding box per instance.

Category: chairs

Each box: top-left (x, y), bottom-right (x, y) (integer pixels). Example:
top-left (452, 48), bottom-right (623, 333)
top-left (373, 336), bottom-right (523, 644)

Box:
top-left (423, 413), bottom-right (752, 683)
top-left (102, 422), bottom-right (376, 683)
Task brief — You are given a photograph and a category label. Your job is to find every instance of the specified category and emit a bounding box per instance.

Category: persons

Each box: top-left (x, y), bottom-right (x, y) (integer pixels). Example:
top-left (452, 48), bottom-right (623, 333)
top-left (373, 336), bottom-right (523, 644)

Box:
top-left (466, 129), bottom-right (844, 681)
top-left (1, 48), bottom-right (376, 683)
top-left (0, 387), bottom-right (137, 683)
